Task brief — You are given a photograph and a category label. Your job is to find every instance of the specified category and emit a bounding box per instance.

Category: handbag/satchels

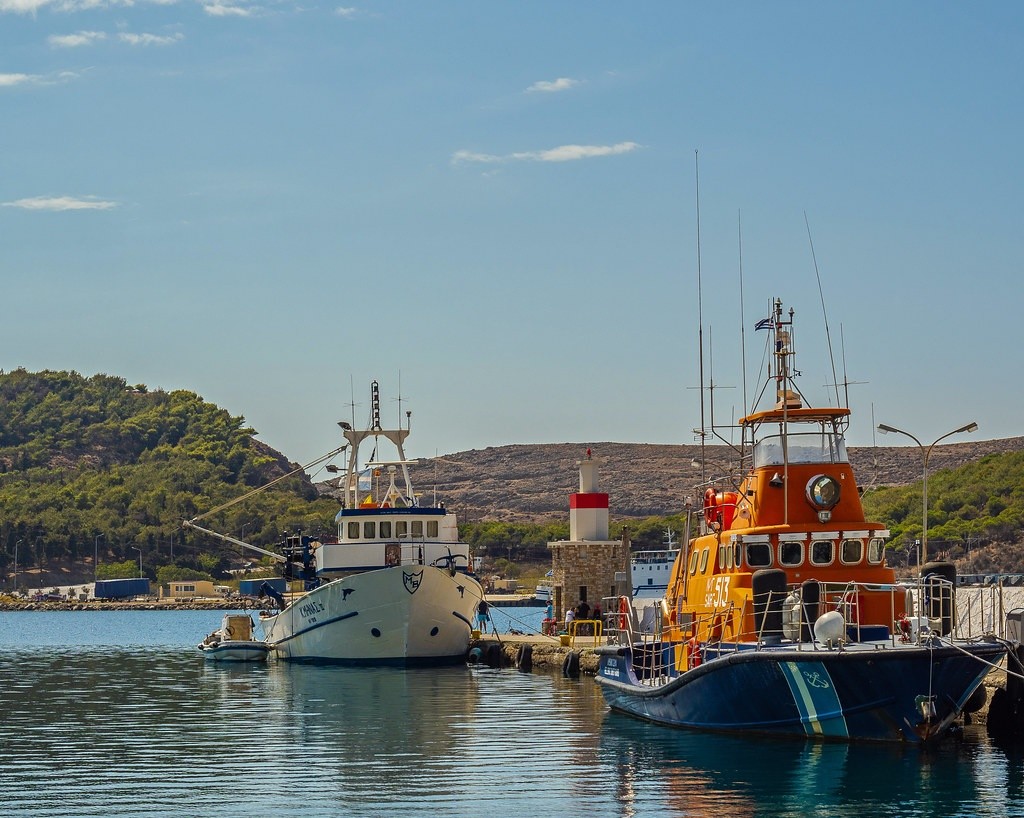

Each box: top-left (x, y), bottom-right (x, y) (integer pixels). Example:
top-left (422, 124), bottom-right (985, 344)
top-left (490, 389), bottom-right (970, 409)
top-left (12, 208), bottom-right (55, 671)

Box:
top-left (486, 614), bottom-right (490, 621)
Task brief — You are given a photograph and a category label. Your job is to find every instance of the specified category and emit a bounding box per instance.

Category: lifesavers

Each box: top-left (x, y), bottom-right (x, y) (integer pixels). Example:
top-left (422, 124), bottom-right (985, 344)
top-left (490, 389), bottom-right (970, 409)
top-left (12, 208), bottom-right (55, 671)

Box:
top-left (516, 644), bottom-right (532, 672)
top-left (686, 638), bottom-right (701, 671)
top-left (619, 597), bottom-right (626, 633)
top-left (703, 488), bottom-right (717, 527)
top-left (562, 652), bottom-right (579, 676)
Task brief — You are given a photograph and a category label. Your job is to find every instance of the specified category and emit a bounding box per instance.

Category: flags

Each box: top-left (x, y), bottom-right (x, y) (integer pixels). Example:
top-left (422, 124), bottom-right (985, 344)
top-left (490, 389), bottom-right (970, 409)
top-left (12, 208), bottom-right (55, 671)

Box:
top-left (339, 468), bottom-right (372, 491)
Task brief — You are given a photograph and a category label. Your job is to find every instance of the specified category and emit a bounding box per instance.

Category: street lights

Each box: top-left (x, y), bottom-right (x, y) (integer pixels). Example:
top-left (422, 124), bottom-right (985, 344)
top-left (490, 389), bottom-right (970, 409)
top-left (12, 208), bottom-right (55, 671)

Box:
top-left (171, 527), bottom-right (182, 563)
top-left (14, 540), bottom-right (22, 591)
top-left (93, 533), bottom-right (104, 583)
top-left (241, 522), bottom-right (250, 568)
top-left (132, 547), bottom-right (143, 578)
top-left (875, 422), bottom-right (981, 629)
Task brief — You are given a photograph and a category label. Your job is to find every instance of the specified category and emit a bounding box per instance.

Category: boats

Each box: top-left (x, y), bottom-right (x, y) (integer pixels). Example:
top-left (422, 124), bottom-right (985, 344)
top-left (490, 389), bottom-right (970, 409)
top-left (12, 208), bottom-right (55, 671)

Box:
top-left (533, 548), bottom-right (681, 602)
top-left (197, 613), bottom-right (269, 662)
top-left (593, 296), bottom-right (1020, 746)
top-left (186, 380), bottom-right (484, 667)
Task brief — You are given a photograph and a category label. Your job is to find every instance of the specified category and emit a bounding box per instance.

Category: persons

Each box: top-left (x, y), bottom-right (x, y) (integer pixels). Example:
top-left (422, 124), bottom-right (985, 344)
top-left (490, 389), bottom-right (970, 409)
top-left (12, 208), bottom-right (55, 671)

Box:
top-left (477, 600), bottom-right (489, 634)
top-left (544, 598), bottom-right (620, 636)
